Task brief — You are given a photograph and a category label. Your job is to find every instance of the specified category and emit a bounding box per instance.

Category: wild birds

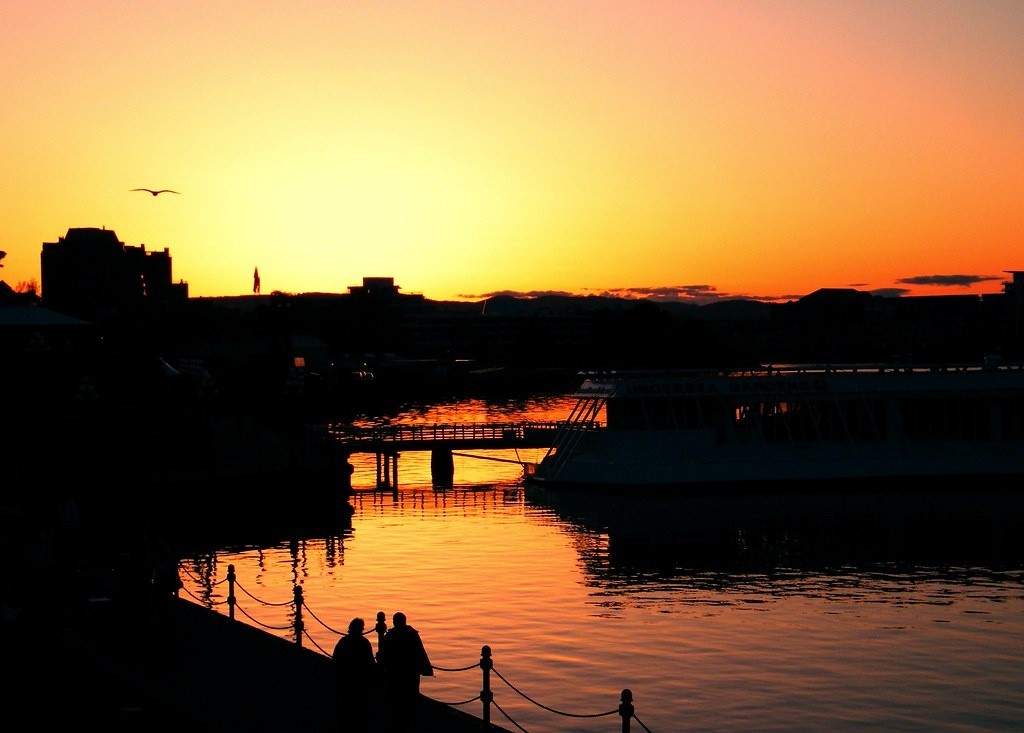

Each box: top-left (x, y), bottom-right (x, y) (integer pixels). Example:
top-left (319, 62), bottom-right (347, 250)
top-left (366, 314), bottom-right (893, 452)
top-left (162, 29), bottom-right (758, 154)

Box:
top-left (129, 188), bottom-right (180, 196)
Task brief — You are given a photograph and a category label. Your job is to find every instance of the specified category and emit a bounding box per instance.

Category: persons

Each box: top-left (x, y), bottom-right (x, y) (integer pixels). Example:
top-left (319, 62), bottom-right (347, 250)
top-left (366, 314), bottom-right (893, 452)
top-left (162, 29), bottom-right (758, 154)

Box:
top-left (57, 492), bottom-right (80, 543)
top-left (377, 613), bottom-right (426, 732)
top-left (334, 619), bottom-right (375, 729)
